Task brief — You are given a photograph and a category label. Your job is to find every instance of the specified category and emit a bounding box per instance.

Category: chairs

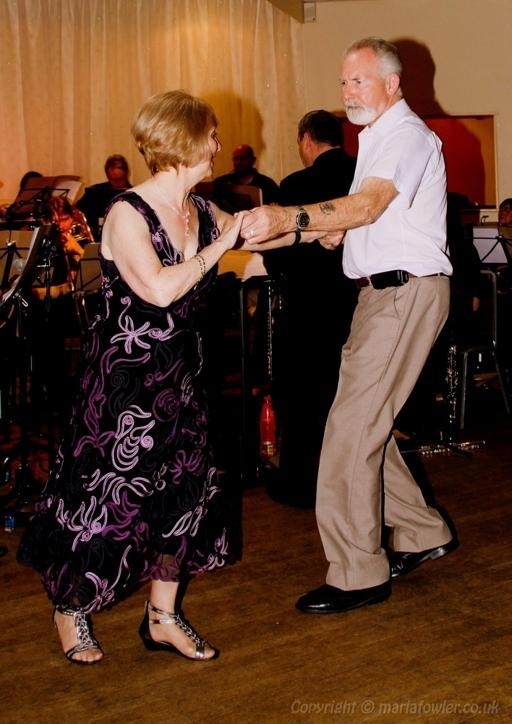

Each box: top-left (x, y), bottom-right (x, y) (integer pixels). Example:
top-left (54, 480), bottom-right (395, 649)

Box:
top-left (456, 268), bottom-right (512, 430)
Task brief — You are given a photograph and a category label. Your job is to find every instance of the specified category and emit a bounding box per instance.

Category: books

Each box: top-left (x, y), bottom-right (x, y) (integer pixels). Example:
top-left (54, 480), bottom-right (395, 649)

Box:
top-left (17, 175), bottom-right (81, 212)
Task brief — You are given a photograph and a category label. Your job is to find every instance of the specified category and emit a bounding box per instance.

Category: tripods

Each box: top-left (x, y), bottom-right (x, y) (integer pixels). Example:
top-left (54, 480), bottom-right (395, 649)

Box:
top-left (0, 305), bottom-right (49, 528)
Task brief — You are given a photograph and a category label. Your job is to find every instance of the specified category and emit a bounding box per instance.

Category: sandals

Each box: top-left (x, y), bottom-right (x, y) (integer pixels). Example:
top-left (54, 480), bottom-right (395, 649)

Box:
top-left (139, 600), bottom-right (219, 662)
top-left (52, 603), bottom-right (102, 666)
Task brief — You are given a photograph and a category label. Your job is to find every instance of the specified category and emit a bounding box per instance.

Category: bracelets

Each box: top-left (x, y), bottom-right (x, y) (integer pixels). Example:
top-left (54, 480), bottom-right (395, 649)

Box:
top-left (293, 228), bottom-right (301, 246)
top-left (197, 254), bottom-right (208, 282)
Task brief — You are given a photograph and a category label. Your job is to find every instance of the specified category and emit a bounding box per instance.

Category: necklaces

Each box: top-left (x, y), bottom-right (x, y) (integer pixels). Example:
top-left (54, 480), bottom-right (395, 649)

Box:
top-left (157, 186), bottom-right (194, 240)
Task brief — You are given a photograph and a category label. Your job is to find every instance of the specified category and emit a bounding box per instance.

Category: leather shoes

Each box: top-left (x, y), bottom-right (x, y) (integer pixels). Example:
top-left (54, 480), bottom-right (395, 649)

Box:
top-left (295, 538), bottom-right (459, 615)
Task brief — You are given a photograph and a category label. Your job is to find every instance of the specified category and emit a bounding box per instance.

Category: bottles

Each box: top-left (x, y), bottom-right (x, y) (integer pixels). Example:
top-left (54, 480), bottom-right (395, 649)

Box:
top-left (258, 394), bottom-right (276, 457)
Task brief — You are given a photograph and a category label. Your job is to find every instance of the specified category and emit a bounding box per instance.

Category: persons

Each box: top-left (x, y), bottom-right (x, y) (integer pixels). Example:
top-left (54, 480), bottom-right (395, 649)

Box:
top-left (5, 172), bottom-right (44, 223)
top-left (497, 197), bottom-right (512, 227)
top-left (225, 144), bottom-right (280, 207)
top-left (234, 35), bottom-right (461, 618)
top-left (266, 109), bottom-right (358, 508)
top-left (30, 201), bottom-right (82, 300)
top-left (76, 155), bottom-right (131, 244)
top-left (445, 183), bottom-right (485, 358)
top-left (15, 92), bottom-right (329, 665)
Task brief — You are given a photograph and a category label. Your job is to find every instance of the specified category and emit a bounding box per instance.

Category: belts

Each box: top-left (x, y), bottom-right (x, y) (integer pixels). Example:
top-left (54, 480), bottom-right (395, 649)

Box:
top-left (357, 269), bottom-right (447, 288)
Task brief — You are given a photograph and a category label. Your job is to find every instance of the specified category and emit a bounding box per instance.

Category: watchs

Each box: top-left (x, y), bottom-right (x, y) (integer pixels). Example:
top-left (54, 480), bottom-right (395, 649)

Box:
top-left (295, 206), bottom-right (310, 231)
